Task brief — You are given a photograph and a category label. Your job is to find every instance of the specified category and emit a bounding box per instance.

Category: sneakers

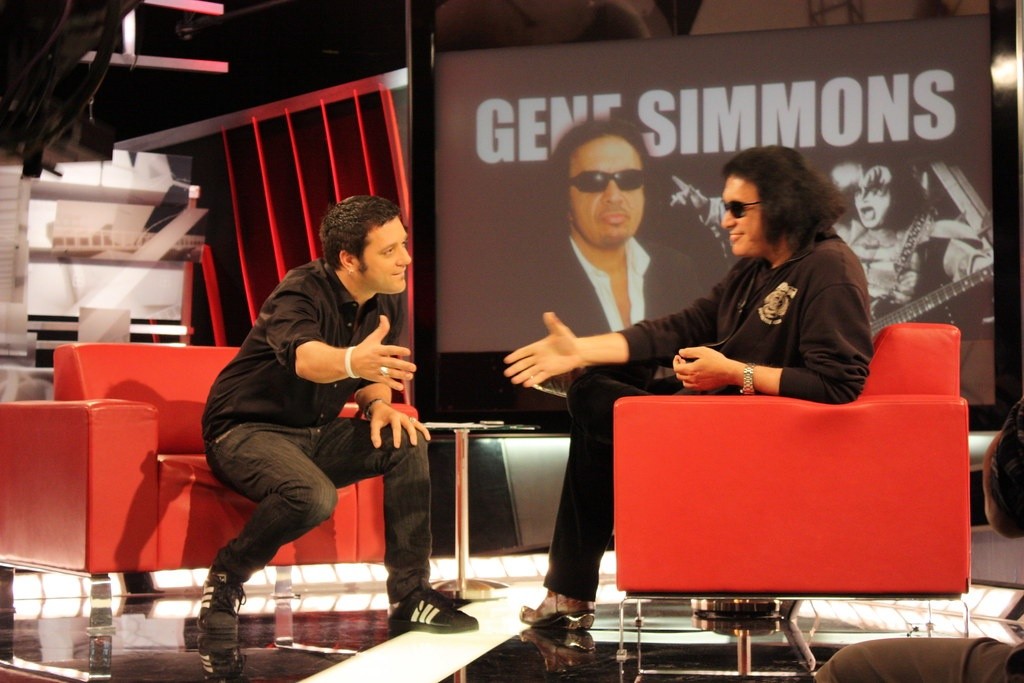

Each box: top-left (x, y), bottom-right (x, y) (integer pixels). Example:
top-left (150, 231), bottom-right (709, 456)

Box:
top-left (196, 565), bottom-right (247, 636)
top-left (196, 632), bottom-right (248, 681)
top-left (387, 579), bottom-right (479, 633)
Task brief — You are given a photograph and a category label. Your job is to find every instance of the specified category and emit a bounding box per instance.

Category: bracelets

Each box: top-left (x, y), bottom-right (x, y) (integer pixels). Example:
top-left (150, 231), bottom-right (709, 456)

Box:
top-left (364, 398), bottom-right (381, 420)
top-left (743, 361), bottom-right (755, 395)
top-left (346, 345), bottom-right (362, 380)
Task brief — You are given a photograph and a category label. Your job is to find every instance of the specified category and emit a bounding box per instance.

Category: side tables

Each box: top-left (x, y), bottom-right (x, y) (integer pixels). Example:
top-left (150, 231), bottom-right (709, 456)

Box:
top-left (418, 421), bottom-right (542, 602)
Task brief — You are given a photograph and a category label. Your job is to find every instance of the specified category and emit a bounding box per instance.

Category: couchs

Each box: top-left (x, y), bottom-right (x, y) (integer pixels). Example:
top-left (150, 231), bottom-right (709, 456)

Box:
top-left (613, 323), bottom-right (972, 677)
top-left (0, 342), bottom-right (419, 624)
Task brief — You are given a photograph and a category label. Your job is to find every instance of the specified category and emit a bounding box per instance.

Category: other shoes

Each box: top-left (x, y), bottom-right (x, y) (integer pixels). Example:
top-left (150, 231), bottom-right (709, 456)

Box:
top-left (519, 624), bottom-right (595, 674)
top-left (518, 587), bottom-right (595, 632)
top-left (690, 597), bottom-right (781, 619)
top-left (689, 613), bottom-right (781, 637)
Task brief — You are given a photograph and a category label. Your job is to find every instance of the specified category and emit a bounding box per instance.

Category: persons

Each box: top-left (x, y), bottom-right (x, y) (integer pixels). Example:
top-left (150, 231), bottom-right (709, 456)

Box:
top-left (666, 151), bottom-right (993, 333)
top-left (527, 117), bottom-right (719, 378)
top-left (503, 143), bottom-right (873, 624)
top-left (198, 196), bottom-right (479, 656)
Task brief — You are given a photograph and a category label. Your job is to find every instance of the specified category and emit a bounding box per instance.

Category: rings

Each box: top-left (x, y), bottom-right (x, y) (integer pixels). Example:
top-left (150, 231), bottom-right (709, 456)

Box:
top-left (380, 368), bottom-right (390, 377)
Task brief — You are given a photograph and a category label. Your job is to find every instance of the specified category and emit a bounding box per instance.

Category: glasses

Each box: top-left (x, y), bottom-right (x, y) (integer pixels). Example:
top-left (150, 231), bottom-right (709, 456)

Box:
top-left (565, 169), bottom-right (647, 193)
top-left (722, 200), bottom-right (761, 218)
